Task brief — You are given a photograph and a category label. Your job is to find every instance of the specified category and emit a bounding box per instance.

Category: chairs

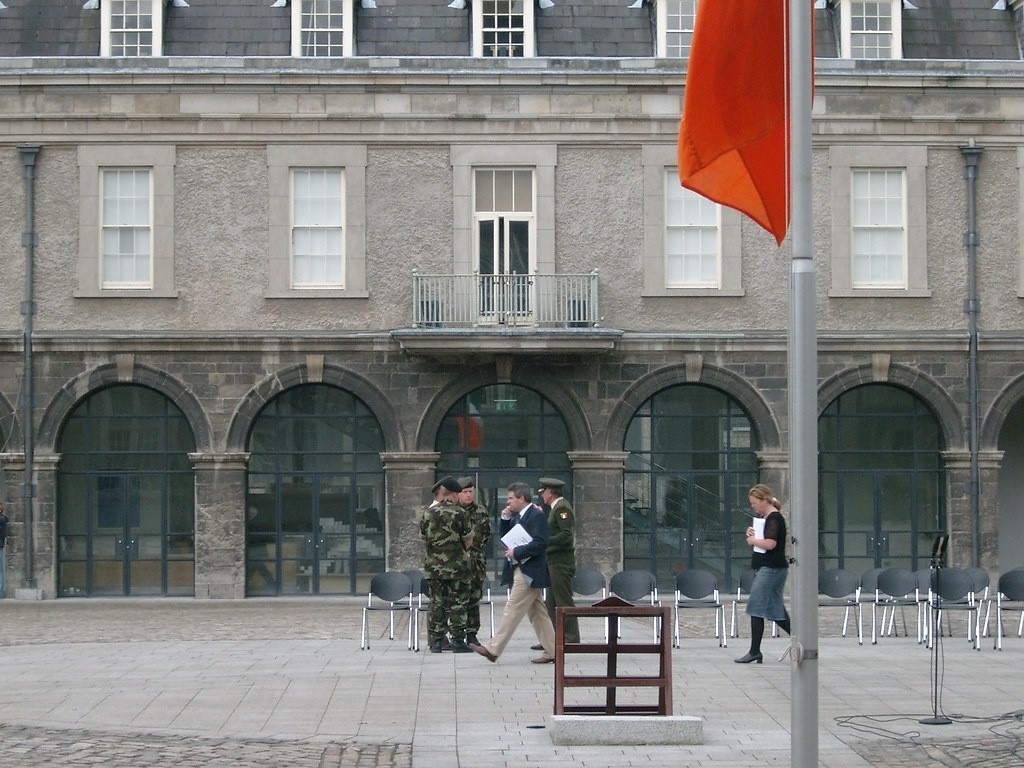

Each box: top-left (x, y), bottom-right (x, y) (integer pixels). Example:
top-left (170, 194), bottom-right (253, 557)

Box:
top-left (361, 568), bottom-right (495, 652)
top-left (818, 567), bottom-right (991, 651)
top-left (993, 566), bottom-right (1024, 651)
top-left (506, 567), bottom-right (662, 643)
top-left (731, 572), bottom-right (780, 639)
top-left (672, 567), bottom-right (727, 651)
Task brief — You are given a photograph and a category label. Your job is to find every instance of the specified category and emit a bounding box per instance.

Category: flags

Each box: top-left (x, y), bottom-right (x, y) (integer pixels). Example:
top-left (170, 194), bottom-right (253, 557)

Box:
top-left (678, 0), bottom-right (815, 249)
top-left (457, 403), bottom-right (485, 448)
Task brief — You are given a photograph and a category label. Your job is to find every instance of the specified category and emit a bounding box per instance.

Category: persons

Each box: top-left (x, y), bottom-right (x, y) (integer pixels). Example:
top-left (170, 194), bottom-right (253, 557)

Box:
top-left (247, 506), bottom-right (275, 585)
top-left (0, 503), bottom-right (9, 597)
top-left (734, 484), bottom-right (791, 663)
top-left (531, 477), bottom-right (581, 649)
top-left (469, 483), bottom-right (555, 663)
top-left (419, 476), bottom-right (490, 653)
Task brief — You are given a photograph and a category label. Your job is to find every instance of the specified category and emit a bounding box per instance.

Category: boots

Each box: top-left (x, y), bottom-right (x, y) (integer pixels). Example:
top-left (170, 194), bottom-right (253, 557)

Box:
top-left (430, 640), bottom-right (442, 653)
top-left (452, 639), bottom-right (474, 652)
top-left (442, 637), bottom-right (453, 649)
top-left (466, 633), bottom-right (480, 646)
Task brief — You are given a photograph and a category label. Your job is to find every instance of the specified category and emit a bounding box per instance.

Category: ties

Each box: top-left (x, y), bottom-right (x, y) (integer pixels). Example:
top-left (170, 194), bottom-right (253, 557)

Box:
top-left (516, 515), bottom-right (521, 525)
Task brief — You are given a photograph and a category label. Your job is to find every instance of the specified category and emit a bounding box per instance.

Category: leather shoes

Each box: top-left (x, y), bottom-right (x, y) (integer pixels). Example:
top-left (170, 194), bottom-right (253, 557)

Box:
top-left (469, 643), bottom-right (495, 662)
top-left (531, 657), bottom-right (556, 664)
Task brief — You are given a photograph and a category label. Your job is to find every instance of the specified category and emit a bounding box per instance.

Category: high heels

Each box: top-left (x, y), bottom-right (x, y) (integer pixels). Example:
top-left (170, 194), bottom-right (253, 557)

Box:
top-left (734, 652), bottom-right (762, 663)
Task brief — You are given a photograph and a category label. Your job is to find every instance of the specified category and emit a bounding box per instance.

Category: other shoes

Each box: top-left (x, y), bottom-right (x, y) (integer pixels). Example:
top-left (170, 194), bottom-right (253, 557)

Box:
top-left (530, 644), bottom-right (544, 651)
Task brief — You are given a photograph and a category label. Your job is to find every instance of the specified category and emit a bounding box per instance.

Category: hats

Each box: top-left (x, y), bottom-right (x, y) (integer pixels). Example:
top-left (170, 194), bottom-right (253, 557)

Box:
top-left (431, 475), bottom-right (452, 495)
top-left (457, 476), bottom-right (472, 489)
top-left (442, 480), bottom-right (462, 493)
top-left (537, 477), bottom-right (566, 492)
